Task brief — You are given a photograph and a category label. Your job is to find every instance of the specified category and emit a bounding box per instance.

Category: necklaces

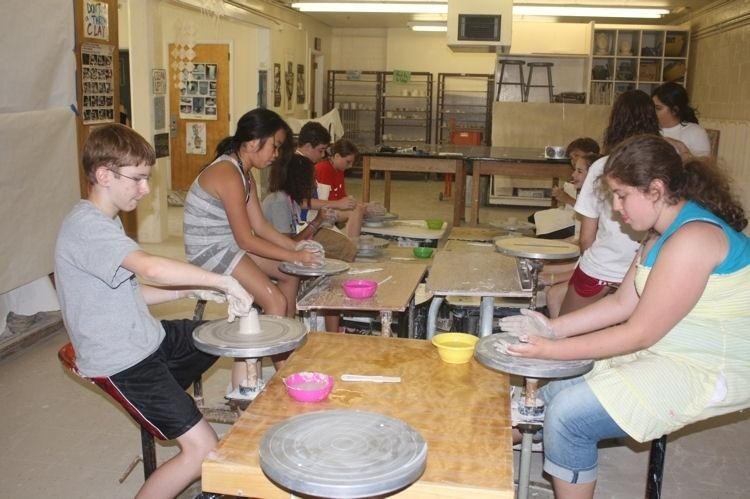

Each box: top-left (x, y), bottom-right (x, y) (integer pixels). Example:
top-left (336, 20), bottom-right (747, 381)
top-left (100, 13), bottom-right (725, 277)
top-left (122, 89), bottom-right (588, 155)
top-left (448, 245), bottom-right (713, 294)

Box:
top-left (234, 150), bottom-right (251, 193)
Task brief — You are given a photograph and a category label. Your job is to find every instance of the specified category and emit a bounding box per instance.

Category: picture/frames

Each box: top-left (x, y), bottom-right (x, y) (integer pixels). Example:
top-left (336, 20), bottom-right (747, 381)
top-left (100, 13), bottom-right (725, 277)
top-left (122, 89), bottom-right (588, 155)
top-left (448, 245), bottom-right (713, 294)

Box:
top-left (119, 57), bottom-right (126, 86)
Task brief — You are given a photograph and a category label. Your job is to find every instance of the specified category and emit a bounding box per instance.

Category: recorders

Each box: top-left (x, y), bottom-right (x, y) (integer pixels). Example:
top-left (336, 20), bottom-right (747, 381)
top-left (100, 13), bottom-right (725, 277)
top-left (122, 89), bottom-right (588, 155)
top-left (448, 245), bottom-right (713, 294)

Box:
top-left (544, 146), bottom-right (570, 159)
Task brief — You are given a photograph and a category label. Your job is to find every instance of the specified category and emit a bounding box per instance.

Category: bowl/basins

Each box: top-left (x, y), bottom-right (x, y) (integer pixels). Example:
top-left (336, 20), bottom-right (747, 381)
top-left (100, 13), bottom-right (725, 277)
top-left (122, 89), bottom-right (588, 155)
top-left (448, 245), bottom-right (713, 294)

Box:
top-left (431, 332), bottom-right (481, 364)
top-left (285, 371), bottom-right (333, 402)
top-left (342, 280), bottom-right (378, 300)
top-left (426, 220), bottom-right (444, 230)
top-left (412, 247), bottom-right (433, 259)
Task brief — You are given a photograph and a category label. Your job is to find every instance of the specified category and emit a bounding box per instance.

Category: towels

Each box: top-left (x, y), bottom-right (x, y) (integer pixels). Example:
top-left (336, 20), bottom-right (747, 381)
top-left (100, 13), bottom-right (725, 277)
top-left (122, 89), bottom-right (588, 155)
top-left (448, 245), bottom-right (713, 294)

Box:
top-left (393, 70), bottom-right (411, 84)
top-left (345, 69), bottom-right (360, 80)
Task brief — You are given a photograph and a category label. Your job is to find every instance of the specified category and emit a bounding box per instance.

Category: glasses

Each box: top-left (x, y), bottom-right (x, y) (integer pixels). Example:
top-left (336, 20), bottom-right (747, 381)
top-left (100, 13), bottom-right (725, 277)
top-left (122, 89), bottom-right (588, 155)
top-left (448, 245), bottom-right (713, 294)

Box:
top-left (107, 167), bottom-right (154, 185)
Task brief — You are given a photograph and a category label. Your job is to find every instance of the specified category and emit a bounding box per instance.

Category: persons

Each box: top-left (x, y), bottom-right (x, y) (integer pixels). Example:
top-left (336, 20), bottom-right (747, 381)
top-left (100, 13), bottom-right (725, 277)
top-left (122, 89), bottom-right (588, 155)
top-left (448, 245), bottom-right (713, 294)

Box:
top-left (314, 140), bottom-right (387, 238)
top-left (493, 134), bottom-right (750, 499)
top-left (184, 109), bottom-right (325, 373)
top-left (52, 123), bottom-right (262, 499)
top-left (597, 34), bottom-right (609, 52)
top-left (620, 38), bottom-right (631, 53)
top-left (261, 154), bottom-right (368, 335)
top-left (294, 122), bottom-right (357, 239)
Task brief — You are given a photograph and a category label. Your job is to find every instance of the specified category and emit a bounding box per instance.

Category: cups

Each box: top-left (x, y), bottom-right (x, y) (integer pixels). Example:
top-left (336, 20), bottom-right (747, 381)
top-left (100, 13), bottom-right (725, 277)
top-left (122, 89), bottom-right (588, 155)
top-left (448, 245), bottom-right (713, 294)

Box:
top-left (330, 100), bottom-right (375, 111)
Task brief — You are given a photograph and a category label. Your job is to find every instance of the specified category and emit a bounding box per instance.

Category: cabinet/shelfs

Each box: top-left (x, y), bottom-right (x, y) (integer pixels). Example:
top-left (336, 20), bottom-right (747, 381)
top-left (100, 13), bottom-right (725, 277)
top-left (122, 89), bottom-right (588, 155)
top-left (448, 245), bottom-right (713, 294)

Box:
top-left (586, 20), bottom-right (692, 108)
top-left (435, 72), bottom-right (495, 181)
top-left (326, 70), bottom-right (381, 179)
top-left (381, 72), bottom-right (433, 181)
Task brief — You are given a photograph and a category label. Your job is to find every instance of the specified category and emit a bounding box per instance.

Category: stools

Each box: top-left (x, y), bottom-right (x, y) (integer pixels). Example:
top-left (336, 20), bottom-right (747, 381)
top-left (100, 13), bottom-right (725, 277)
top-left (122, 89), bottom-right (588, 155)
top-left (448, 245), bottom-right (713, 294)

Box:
top-left (57, 342), bottom-right (221, 481)
top-left (192, 299), bottom-right (262, 321)
top-left (624, 407), bottom-right (749, 499)
top-left (496, 60), bottom-right (526, 102)
top-left (525, 62), bottom-right (555, 103)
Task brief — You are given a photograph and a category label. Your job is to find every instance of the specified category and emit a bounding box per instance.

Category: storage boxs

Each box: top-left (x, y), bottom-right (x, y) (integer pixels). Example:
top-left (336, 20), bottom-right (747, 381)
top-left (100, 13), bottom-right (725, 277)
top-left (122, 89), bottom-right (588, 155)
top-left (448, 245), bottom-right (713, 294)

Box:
top-left (450, 130), bottom-right (483, 146)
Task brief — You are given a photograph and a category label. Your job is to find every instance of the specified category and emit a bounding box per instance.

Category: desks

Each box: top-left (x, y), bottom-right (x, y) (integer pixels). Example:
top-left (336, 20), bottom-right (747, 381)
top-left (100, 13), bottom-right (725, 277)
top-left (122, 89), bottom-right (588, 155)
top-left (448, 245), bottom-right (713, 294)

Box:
top-left (358, 140), bottom-right (473, 227)
top-left (293, 219), bottom-right (547, 340)
top-left (467, 146), bottom-right (576, 225)
top-left (201, 331), bottom-right (515, 499)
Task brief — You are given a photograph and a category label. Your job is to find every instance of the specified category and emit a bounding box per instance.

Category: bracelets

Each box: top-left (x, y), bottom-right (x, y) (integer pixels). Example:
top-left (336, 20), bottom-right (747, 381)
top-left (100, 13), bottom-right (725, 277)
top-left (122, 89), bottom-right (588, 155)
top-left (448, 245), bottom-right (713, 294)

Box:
top-left (308, 223), bottom-right (317, 233)
